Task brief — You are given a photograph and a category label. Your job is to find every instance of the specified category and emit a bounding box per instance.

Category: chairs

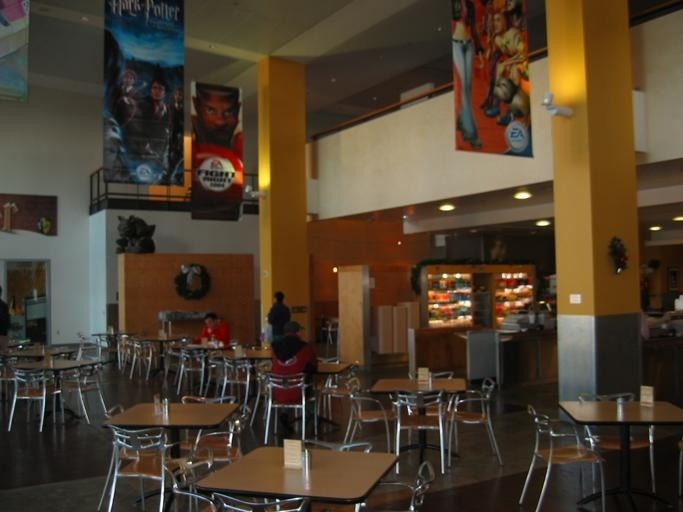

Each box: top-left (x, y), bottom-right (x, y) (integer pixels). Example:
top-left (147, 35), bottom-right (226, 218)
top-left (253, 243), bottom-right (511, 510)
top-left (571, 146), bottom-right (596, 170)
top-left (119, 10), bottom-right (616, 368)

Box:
top-left (580, 391), bottom-right (656, 495)
top-left (517, 406), bottom-right (604, 511)
top-left (1, 314), bottom-right (505, 511)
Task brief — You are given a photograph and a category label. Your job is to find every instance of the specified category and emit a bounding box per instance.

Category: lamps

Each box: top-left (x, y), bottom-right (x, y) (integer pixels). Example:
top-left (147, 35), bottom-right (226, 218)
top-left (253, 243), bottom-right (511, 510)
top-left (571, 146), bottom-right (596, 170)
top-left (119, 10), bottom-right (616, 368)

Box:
top-left (515, 185), bottom-right (532, 200)
top-left (540, 88), bottom-right (574, 119)
top-left (242, 183), bottom-right (266, 201)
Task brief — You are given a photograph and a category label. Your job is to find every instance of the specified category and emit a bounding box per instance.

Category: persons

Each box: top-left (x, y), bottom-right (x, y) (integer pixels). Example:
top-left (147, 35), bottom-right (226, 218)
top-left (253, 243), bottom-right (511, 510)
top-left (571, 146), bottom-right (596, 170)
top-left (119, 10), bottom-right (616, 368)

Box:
top-left (266, 319), bottom-right (323, 430)
top-left (190, 312), bottom-right (231, 400)
top-left (0, 285), bottom-right (12, 352)
top-left (663, 293), bottom-right (683, 325)
top-left (640, 301), bottom-right (671, 343)
top-left (263, 288), bottom-right (292, 342)
top-left (452, 0), bottom-right (530, 150)
top-left (191, 80), bottom-right (243, 160)
top-left (103, 69), bottom-right (184, 181)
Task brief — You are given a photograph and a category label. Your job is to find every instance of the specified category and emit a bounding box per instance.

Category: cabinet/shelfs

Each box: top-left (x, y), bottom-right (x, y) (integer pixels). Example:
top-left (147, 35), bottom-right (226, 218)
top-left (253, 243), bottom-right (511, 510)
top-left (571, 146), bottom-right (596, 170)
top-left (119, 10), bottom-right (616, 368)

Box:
top-left (484, 264), bottom-right (538, 325)
top-left (421, 264), bottom-right (480, 330)
